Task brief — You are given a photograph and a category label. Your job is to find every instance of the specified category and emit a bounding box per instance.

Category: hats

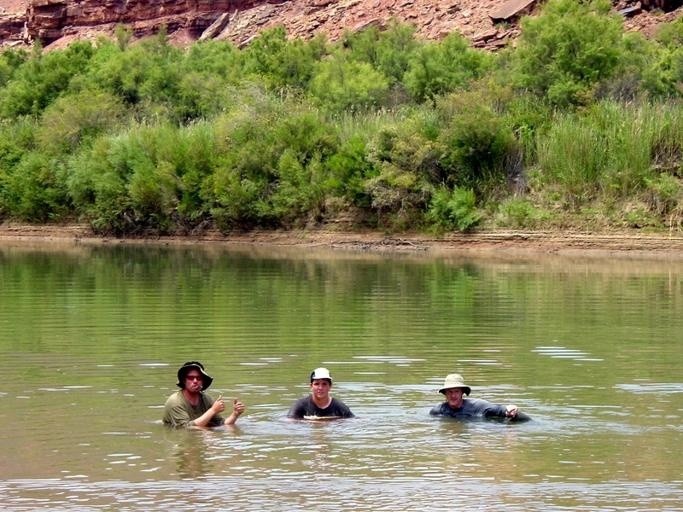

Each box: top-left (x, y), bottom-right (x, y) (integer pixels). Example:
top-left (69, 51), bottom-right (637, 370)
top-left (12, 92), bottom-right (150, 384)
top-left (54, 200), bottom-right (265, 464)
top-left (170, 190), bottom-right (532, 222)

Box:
top-left (308, 366), bottom-right (332, 383)
top-left (176, 360), bottom-right (213, 392)
top-left (437, 372), bottom-right (472, 396)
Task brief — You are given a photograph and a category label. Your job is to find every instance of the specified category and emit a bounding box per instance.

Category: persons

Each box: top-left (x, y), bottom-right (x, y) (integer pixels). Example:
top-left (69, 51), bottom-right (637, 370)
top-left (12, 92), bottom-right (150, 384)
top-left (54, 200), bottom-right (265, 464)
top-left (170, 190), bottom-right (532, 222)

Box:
top-left (163, 426), bottom-right (241, 480)
top-left (290, 419), bottom-right (341, 468)
top-left (287, 365), bottom-right (354, 418)
top-left (426, 371), bottom-right (530, 425)
top-left (160, 360), bottom-right (245, 427)
